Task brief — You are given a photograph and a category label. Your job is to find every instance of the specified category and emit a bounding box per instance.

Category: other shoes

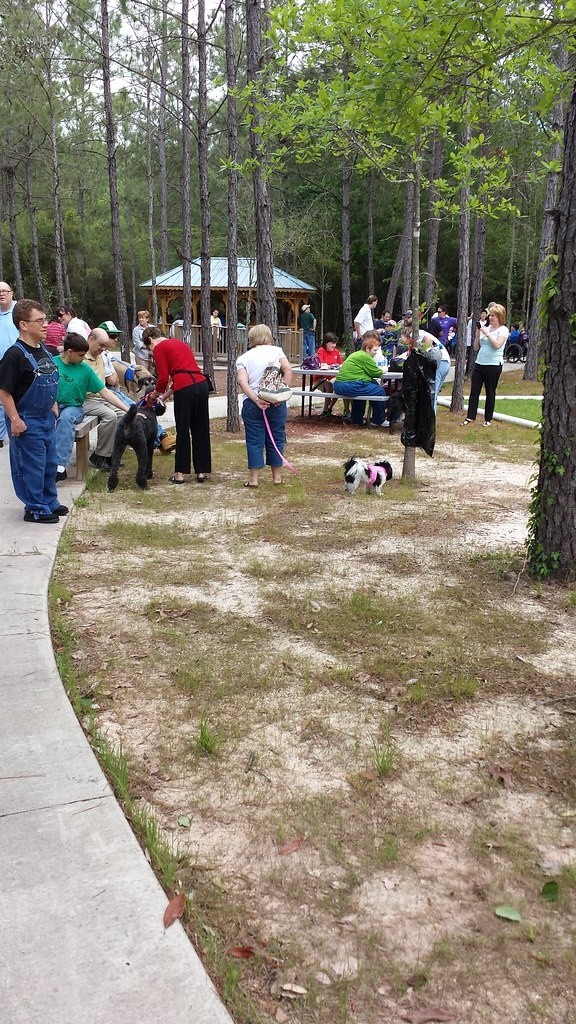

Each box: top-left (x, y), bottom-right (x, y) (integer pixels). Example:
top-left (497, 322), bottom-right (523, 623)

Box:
top-left (55, 469), bottom-right (68, 484)
top-left (159, 432), bottom-right (177, 455)
top-left (89, 451), bottom-right (124, 472)
top-left (24, 504), bottom-right (68, 523)
top-left (342, 409), bottom-right (351, 420)
top-left (172, 473), bottom-right (185, 484)
top-left (370, 419), bottom-right (390, 427)
top-left (196, 473), bottom-right (207, 483)
top-left (323, 409), bottom-right (332, 417)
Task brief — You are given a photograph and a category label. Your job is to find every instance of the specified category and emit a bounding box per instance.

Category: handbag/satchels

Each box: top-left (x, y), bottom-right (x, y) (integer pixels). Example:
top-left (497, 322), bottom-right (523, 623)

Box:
top-left (387, 357), bottom-right (406, 372)
top-left (203, 373), bottom-right (214, 391)
top-left (300, 355), bottom-right (322, 370)
top-left (257, 366), bottom-right (293, 403)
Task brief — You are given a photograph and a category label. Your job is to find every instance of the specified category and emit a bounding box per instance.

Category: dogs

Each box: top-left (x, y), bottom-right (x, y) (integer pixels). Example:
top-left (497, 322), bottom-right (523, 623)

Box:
top-left (342, 453), bottom-right (392, 497)
top-left (107, 385), bottom-right (166, 491)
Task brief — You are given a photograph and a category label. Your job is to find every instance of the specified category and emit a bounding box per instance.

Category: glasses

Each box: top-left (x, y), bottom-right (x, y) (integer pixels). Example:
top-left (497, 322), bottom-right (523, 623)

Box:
top-left (19, 316), bottom-right (51, 325)
top-left (57, 312), bottom-right (66, 317)
top-left (108, 333), bottom-right (120, 339)
top-left (437, 311), bottom-right (445, 313)
top-left (0, 289), bottom-right (11, 295)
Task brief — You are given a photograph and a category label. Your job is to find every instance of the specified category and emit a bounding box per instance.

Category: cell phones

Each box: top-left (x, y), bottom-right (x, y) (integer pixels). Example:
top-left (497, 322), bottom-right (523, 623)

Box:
top-left (476, 322), bottom-right (481, 329)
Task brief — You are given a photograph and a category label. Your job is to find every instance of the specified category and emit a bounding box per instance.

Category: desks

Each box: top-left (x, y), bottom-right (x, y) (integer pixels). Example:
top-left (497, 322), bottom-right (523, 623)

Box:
top-left (291, 367), bottom-right (404, 435)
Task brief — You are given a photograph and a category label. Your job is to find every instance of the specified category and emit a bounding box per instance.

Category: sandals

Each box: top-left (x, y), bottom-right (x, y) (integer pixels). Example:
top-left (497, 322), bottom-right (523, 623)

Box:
top-left (482, 421), bottom-right (492, 427)
top-left (460, 418), bottom-right (474, 426)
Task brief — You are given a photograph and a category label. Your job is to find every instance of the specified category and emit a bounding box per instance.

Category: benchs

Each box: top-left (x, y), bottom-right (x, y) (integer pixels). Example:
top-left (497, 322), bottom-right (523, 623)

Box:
top-left (55, 414), bottom-right (102, 485)
top-left (292, 388), bottom-right (391, 402)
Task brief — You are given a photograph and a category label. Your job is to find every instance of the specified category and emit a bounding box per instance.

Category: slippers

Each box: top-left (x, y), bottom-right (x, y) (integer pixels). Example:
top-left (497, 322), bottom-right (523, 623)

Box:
top-left (244, 481), bottom-right (258, 488)
top-left (274, 480), bottom-right (285, 485)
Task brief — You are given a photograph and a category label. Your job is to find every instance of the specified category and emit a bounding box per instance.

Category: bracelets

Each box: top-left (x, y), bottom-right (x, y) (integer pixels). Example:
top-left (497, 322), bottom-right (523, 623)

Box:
top-left (255, 398), bottom-right (259, 403)
top-left (486, 334), bottom-right (491, 336)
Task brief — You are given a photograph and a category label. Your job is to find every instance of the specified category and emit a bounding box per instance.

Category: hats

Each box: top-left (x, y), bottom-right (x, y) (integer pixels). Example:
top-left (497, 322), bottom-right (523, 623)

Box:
top-left (384, 319), bottom-right (396, 326)
top-left (301, 304), bottom-right (310, 311)
top-left (402, 310), bottom-right (413, 317)
top-left (98, 320), bottom-right (123, 333)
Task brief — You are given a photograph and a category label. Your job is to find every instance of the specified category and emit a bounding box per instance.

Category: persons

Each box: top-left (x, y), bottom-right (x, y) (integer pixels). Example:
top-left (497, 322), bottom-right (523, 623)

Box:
top-left (235, 325), bottom-right (293, 489)
top-left (0, 282), bottom-right (225, 485)
top-left (0, 299), bottom-right (70, 524)
top-left (312, 332), bottom-right (351, 419)
top-left (298, 304), bottom-right (317, 361)
top-left (333, 294), bottom-right (527, 427)
